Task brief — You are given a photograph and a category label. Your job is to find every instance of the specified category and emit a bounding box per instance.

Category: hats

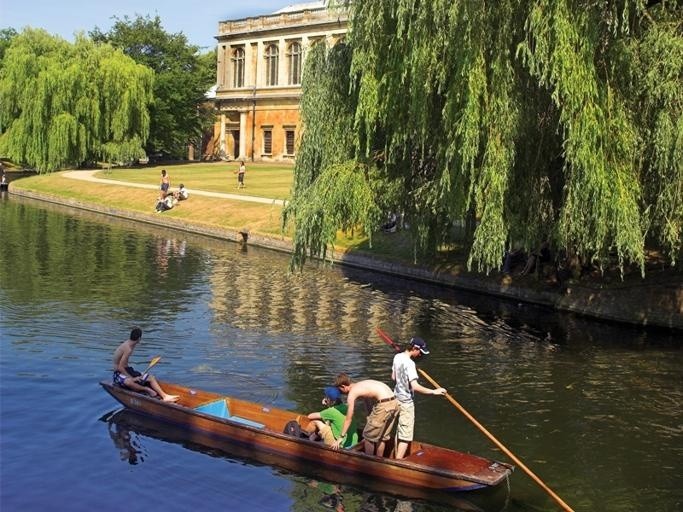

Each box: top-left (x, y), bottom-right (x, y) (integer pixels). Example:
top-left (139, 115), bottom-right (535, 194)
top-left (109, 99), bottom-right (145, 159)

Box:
top-left (323, 386), bottom-right (341, 401)
top-left (410, 336), bottom-right (430, 355)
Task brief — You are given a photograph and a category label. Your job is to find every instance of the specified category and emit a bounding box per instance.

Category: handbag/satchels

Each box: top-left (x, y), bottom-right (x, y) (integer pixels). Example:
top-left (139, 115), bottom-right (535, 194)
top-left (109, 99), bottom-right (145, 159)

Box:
top-left (284, 421), bottom-right (308, 439)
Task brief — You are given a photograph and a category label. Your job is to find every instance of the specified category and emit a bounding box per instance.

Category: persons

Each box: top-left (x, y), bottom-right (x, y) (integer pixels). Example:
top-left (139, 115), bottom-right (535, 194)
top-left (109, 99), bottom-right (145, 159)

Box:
top-left (305, 387), bottom-right (359, 448)
top-left (112, 328), bottom-right (181, 402)
top-left (330, 372), bottom-right (398, 458)
top-left (154, 170), bottom-right (188, 213)
top-left (235, 161), bottom-right (247, 189)
top-left (509, 235), bottom-right (582, 283)
top-left (390, 336), bottom-right (447, 461)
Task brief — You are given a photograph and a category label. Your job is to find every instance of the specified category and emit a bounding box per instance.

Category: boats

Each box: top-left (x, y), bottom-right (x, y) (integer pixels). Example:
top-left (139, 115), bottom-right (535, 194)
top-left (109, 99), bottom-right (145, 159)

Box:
top-left (99, 377), bottom-right (515, 493)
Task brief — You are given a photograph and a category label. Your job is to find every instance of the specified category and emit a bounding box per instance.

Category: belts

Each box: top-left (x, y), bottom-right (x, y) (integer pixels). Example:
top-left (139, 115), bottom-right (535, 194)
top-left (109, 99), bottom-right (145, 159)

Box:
top-left (378, 396), bottom-right (396, 402)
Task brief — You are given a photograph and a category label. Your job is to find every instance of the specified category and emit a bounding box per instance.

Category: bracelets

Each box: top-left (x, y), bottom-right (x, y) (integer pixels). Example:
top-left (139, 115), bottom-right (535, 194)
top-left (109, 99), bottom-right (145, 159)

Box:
top-left (340, 434), bottom-right (345, 438)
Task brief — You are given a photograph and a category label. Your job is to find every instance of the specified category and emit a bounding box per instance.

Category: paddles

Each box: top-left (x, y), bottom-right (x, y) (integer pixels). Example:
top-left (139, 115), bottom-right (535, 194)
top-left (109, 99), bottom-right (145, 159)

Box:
top-left (140, 357), bottom-right (162, 376)
top-left (377, 327), bottom-right (582, 511)
